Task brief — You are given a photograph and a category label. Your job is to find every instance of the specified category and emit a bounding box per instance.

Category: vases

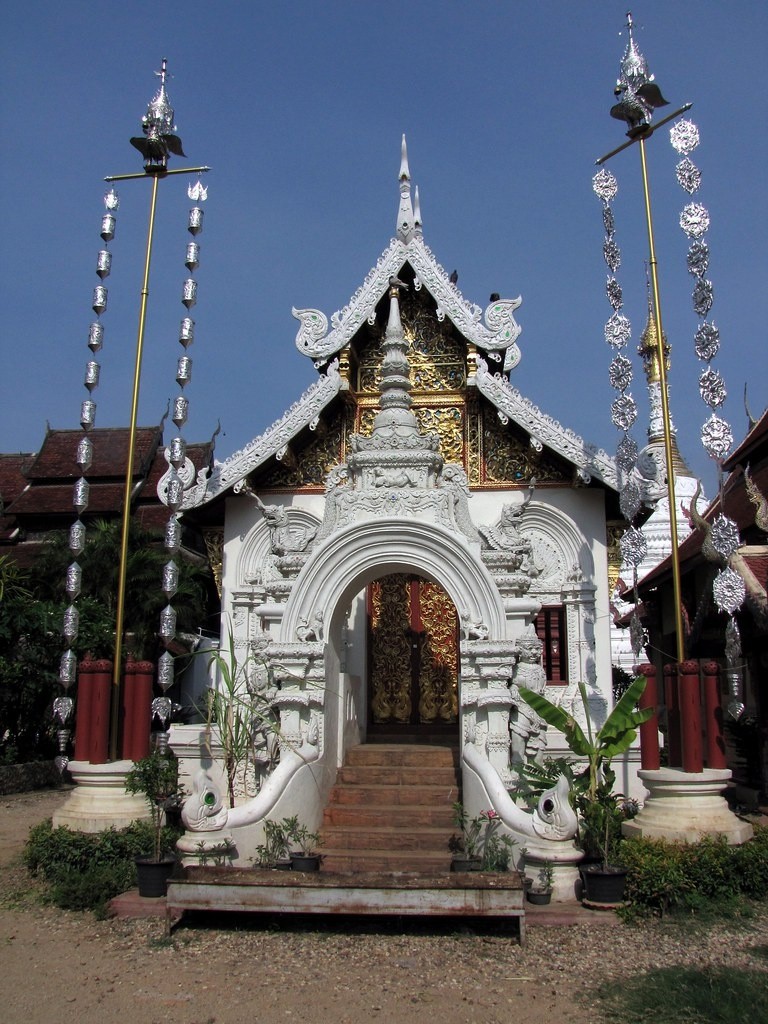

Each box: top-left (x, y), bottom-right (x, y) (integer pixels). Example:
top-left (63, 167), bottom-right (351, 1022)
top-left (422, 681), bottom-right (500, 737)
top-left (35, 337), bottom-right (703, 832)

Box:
top-left (450, 855), bottom-right (481, 872)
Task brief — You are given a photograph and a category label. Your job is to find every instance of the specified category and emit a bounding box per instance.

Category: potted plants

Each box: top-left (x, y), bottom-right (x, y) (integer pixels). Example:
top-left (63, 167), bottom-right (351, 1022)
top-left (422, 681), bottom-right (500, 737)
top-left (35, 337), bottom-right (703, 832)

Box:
top-left (527, 857), bottom-right (557, 904)
top-left (283, 815), bottom-right (327, 872)
top-left (127, 756), bottom-right (182, 899)
top-left (507, 671), bottom-right (655, 884)
top-left (259, 821), bottom-right (292, 872)
top-left (578, 799), bottom-right (628, 903)
top-left (521, 848), bottom-right (534, 895)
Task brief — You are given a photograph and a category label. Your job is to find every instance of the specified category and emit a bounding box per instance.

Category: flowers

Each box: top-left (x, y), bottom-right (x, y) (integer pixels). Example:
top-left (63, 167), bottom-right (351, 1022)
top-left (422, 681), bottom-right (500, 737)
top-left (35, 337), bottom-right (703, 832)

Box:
top-left (445, 802), bottom-right (503, 861)
